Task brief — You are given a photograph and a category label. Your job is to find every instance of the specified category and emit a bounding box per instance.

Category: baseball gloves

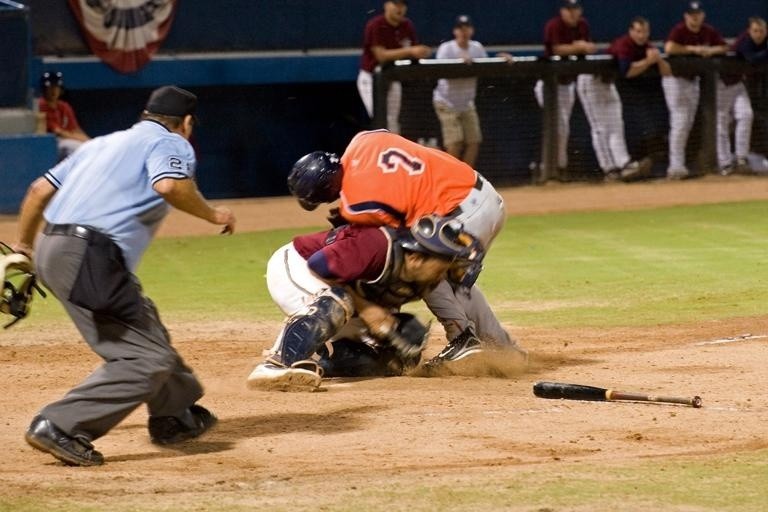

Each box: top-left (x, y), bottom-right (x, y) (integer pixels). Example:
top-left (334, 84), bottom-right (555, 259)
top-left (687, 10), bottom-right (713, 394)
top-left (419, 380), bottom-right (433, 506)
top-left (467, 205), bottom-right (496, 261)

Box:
top-left (372, 313), bottom-right (426, 372)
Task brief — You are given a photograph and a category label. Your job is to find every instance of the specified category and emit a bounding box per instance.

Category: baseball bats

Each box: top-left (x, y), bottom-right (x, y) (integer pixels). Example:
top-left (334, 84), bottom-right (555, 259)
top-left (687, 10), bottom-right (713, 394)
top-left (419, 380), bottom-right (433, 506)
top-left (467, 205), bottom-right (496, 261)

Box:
top-left (534, 382), bottom-right (702, 408)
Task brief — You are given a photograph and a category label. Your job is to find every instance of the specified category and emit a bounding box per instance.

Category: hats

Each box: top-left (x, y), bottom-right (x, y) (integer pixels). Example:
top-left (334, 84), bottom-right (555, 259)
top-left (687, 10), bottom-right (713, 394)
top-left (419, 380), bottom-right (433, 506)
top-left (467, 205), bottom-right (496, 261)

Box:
top-left (145, 85), bottom-right (199, 117)
top-left (683, 1), bottom-right (706, 14)
top-left (559, 0), bottom-right (581, 9)
top-left (453, 14), bottom-right (472, 27)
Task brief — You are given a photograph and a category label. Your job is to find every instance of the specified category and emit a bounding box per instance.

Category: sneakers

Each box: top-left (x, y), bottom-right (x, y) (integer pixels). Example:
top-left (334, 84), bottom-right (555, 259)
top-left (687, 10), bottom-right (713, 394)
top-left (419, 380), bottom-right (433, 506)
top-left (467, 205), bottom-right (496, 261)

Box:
top-left (619, 155), bottom-right (654, 181)
top-left (421, 326), bottom-right (485, 369)
top-left (245, 364), bottom-right (322, 392)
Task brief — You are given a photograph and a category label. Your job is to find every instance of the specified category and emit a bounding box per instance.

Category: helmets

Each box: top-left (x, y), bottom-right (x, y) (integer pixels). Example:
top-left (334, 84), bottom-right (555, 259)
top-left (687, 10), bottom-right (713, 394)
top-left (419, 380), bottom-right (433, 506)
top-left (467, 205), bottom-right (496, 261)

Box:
top-left (286, 150), bottom-right (343, 211)
top-left (395, 214), bottom-right (485, 300)
top-left (40, 70), bottom-right (64, 96)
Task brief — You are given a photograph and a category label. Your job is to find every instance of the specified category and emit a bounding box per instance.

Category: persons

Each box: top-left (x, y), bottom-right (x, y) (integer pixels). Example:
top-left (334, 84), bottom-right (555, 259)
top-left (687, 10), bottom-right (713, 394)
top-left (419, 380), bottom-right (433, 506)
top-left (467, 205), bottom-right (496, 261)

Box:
top-left (11, 85), bottom-right (239, 467)
top-left (241, 212), bottom-right (473, 395)
top-left (357, 1), bottom-right (432, 136)
top-left (433, 15), bottom-right (515, 170)
top-left (715, 16), bottom-right (768, 178)
top-left (662, 2), bottom-right (731, 179)
top-left (283, 126), bottom-right (532, 366)
top-left (534, 1), bottom-right (598, 181)
top-left (575, 17), bottom-right (672, 181)
top-left (40, 68), bottom-right (89, 152)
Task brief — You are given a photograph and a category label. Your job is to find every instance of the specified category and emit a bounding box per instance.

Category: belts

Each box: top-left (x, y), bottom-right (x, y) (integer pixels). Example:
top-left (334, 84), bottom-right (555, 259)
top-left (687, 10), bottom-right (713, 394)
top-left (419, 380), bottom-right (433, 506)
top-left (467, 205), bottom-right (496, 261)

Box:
top-left (442, 173), bottom-right (484, 220)
top-left (42, 222), bottom-right (122, 260)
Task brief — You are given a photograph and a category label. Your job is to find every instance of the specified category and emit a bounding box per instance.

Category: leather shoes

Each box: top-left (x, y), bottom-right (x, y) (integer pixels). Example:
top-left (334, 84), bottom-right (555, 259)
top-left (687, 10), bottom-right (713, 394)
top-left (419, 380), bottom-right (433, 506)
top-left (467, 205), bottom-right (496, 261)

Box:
top-left (148, 405), bottom-right (217, 445)
top-left (25, 414), bottom-right (104, 466)
